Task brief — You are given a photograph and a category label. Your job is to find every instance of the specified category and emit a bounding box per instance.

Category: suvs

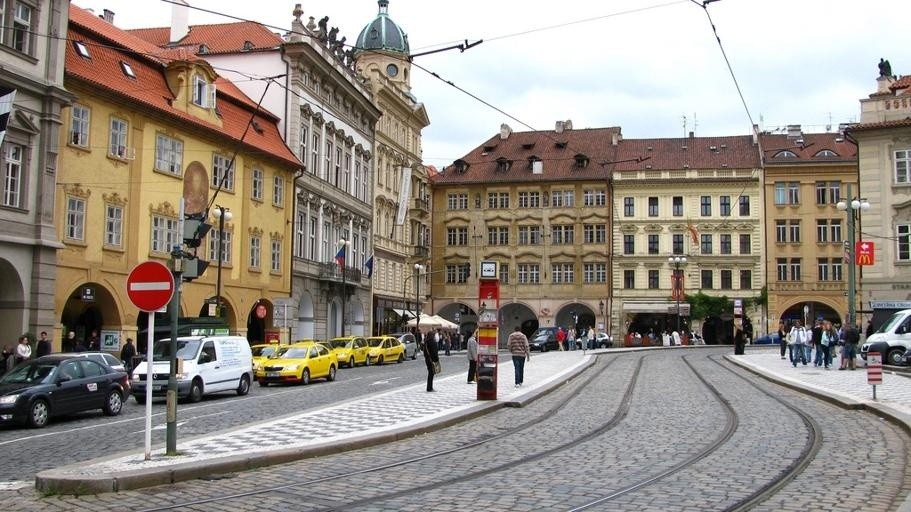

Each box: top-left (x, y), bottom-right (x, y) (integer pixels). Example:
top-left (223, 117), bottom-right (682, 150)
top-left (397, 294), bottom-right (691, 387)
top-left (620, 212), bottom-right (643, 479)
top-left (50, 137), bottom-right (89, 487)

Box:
top-left (527, 326), bottom-right (560, 353)
top-left (575, 332), bottom-right (613, 349)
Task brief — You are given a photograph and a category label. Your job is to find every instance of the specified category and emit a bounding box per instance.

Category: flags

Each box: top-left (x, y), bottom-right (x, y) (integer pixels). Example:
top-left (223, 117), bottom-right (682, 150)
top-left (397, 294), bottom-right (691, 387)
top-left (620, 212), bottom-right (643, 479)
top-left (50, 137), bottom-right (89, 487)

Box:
top-left (335, 244), bottom-right (343, 272)
top-left (365, 255), bottom-right (372, 279)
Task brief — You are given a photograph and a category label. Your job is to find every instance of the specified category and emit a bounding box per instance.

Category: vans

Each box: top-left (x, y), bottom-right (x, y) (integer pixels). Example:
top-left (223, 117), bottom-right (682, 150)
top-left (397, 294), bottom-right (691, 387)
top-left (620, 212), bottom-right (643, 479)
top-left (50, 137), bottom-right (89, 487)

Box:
top-left (388, 331), bottom-right (418, 360)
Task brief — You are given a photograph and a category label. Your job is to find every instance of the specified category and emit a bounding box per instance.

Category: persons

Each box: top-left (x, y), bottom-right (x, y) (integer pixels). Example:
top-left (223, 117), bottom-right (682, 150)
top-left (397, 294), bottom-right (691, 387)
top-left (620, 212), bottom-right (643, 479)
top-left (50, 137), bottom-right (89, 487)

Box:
top-left (557, 325), bottom-right (685, 351)
top-left (121, 338), bottom-right (136, 380)
top-left (415, 329), bottom-right (465, 356)
top-left (84, 330), bottom-right (100, 351)
top-left (2, 344), bottom-right (14, 371)
top-left (36, 331), bottom-right (52, 357)
top-left (778, 319), bottom-right (860, 371)
top-left (423, 331), bottom-right (441, 392)
top-left (466, 329), bottom-right (477, 384)
top-left (507, 326), bottom-right (530, 388)
top-left (16, 335), bottom-right (32, 362)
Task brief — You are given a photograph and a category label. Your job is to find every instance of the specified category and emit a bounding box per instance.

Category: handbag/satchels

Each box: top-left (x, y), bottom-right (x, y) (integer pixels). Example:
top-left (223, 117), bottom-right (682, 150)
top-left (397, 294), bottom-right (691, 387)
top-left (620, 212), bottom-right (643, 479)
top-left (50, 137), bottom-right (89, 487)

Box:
top-left (433, 361), bottom-right (442, 374)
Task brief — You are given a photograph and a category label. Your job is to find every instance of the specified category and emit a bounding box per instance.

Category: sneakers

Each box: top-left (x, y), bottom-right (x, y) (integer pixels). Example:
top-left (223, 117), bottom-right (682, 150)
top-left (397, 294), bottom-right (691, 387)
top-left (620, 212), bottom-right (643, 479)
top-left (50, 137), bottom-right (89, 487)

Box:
top-left (468, 381), bottom-right (477, 383)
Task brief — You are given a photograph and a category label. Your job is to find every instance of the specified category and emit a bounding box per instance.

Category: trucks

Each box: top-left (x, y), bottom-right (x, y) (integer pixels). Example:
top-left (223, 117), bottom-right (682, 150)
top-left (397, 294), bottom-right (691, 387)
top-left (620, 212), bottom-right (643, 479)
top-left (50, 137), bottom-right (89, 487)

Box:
top-left (132, 335), bottom-right (255, 402)
top-left (860, 309), bottom-right (910, 366)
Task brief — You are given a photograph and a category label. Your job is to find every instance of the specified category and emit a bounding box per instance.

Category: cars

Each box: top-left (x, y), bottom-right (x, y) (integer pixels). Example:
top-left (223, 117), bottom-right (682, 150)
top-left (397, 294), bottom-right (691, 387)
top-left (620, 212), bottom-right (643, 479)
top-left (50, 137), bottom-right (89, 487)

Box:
top-left (901, 345), bottom-right (910, 365)
top-left (363, 334), bottom-right (405, 365)
top-left (752, 331), bottom-right (779, 344)
top-left (328, 335), bottom-right (372, 367)
top-left (19, 351), bottom-right (133, 399)
top-left (0, 356), bottom-right (130, 427)
top-left (298, 339), bottom-right (339, 368)
top-left (250, 340), bottom-right (291, 377)
top-left (256, 341), bottom-right (339, 385)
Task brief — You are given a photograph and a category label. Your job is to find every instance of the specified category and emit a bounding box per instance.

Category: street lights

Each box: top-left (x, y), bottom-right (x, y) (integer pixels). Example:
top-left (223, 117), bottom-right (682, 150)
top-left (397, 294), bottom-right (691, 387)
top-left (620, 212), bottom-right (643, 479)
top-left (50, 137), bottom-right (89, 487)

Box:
top-left (667, 255), bottom-right (687, 336)
top-left (837, 196), bottom-right (870, 334)
top-left (207, 202), bottom-right (231, 321)
top-left (414, 262), bottom-right (424, 335)
top-left (339, 238), bottom-right (351, 336)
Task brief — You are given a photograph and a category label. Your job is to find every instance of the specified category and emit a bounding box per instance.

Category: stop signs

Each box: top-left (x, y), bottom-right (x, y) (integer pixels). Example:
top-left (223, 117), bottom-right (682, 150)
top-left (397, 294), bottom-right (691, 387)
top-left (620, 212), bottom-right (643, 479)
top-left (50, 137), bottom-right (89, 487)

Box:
top-left (125, 262), bottom-right (178, 314)
